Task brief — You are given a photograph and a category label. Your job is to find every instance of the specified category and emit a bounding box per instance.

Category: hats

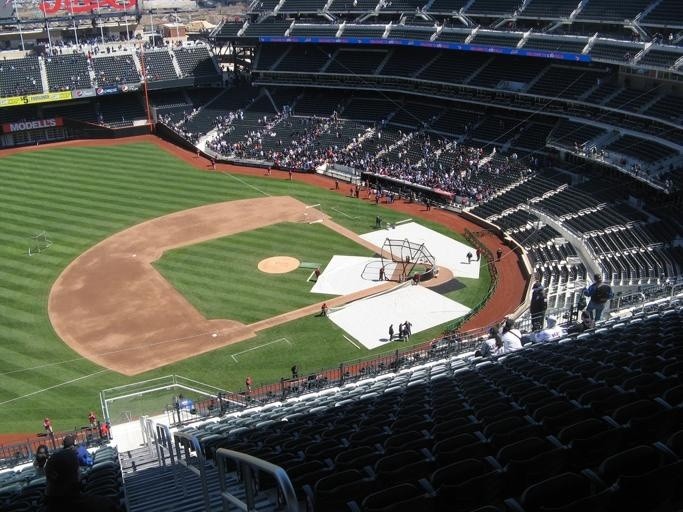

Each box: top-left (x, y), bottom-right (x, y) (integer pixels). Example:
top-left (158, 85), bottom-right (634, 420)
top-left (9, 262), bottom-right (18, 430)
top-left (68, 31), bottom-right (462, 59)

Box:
top-left (488, 327), bottom-right (499, 334)
top-left (532, 283), bottom-right (544, 290)
top-left (37, 444), bottom-right (48, 454)
top-left (63, 434), bottom-right (77, 447)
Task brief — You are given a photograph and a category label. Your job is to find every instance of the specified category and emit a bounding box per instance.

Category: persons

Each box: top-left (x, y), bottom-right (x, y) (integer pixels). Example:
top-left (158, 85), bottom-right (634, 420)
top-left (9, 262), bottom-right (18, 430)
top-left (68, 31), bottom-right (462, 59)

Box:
top-left (467, 251), bottom-right (472, 264)
top-left (496, 248), bottom-right (502, 261)
top-left (314, 268), bottom-right (320, 278)
top-left (2, 6), bottom-right (682, 209)
top-left (379, 266), bottom-right (385, 280)
top-left (14, 271), bottom-right (617, 501)
top-left (376, 216), bottom-right (382, 227)
top-left (476, 248), bottom-right (481, 262)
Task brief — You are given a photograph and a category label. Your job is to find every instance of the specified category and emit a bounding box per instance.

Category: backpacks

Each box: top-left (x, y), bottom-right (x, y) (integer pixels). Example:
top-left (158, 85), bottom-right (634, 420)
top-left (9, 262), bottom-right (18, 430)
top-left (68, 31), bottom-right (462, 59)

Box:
top-left (594, 284), bottom-right (611, 303)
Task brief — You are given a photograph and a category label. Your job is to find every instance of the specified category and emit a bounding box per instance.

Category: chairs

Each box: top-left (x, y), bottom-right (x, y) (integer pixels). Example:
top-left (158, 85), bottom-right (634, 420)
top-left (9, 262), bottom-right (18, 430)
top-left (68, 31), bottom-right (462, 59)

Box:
top-left (464, 171), bottom-right (683, 307)
